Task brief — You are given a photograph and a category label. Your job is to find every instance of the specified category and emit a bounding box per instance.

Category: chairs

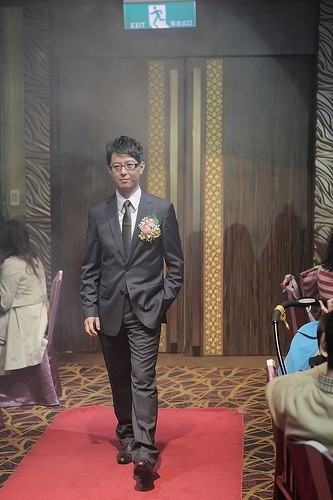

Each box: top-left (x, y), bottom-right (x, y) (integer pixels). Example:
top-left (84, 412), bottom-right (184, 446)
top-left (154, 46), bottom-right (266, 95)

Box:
top-left (1, 270), bottom-right (63, 406)
top-left (265, 274), bottom-right (333, 500)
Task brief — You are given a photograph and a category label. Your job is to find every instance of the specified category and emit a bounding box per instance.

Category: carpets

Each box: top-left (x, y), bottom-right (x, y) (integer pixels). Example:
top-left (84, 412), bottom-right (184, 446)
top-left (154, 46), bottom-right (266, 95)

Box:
top-left (0, 406), bottom-right (244, 500)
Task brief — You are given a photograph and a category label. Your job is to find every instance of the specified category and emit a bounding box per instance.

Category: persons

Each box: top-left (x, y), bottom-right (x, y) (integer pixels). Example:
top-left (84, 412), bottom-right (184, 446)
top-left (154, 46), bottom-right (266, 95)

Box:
top-left (79, 136), bottom-right (185, 479)
top-left (316, 229), bottom-right (333, 314)
top-left (0, 219), bottom-right (49, 376)
top-left (262, 311), bottom-right (333, 469)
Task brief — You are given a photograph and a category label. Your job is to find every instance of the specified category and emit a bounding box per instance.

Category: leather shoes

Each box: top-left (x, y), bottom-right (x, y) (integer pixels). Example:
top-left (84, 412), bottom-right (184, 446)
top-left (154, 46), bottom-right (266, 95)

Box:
top-left (134, 456), bottom-right (157, 490)
top-left (116, 442), bottom-right (134, 465)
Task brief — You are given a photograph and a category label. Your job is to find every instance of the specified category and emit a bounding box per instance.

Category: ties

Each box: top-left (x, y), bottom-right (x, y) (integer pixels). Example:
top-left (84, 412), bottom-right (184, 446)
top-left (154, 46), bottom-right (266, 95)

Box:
top-left (123, 202), bottom-right (131, 257)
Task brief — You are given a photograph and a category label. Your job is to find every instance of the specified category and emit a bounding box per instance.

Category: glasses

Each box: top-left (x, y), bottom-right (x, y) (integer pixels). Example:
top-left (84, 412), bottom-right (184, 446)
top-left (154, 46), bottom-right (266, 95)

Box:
top-left (107, 160), bottom-right (142, 172)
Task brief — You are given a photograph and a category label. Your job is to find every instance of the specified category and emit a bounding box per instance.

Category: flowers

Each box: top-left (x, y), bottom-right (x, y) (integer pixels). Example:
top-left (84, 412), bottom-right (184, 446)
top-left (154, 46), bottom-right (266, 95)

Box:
top-left (138, 215), bottom-right (161, 244)
